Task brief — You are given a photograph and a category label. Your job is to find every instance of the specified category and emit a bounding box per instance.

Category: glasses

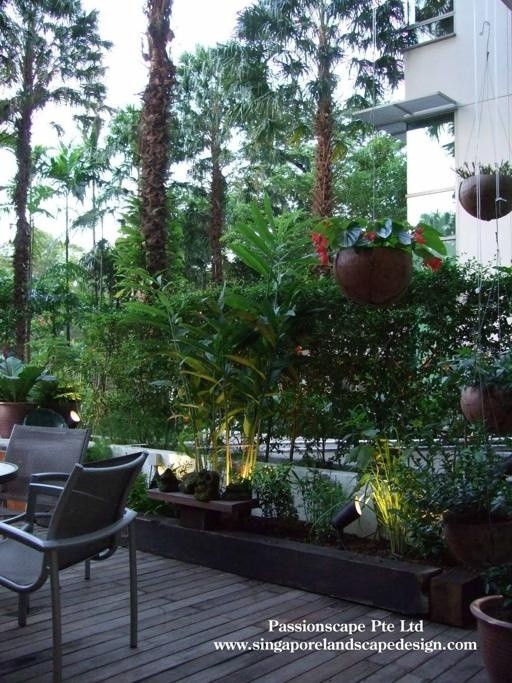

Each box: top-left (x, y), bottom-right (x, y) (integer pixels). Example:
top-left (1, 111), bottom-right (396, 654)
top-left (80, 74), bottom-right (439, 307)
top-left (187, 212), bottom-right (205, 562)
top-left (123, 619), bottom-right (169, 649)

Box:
top-left (65, 408), bottom-right (81, 430)
top-left (328, 494), bottom-right (364, 553)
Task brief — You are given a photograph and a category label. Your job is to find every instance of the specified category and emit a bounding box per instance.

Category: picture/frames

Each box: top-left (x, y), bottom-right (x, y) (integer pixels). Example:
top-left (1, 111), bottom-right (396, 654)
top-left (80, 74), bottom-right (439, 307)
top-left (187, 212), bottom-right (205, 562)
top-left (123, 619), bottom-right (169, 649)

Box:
top-left (0, 460), bottom-right (21, 543)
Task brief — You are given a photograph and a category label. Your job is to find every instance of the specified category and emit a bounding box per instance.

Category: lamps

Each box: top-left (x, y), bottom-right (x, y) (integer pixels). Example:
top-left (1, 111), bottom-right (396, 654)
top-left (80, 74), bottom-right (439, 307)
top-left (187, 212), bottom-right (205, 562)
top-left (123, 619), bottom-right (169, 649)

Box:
top-left (1, 418), bottom-right (93, 611)
top-left (1, 450), bottom-right (153, 682)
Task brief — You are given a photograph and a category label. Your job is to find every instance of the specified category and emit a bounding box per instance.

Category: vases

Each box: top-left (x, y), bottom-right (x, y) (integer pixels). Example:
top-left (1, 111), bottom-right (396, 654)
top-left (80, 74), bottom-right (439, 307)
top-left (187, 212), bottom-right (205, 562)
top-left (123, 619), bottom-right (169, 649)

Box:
top-left (300, 209), bottom-right (452, 274)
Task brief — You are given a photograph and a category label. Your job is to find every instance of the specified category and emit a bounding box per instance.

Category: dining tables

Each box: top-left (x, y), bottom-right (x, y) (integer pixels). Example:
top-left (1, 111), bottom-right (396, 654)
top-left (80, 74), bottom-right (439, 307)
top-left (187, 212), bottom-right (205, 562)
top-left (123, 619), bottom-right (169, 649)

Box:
top-left (450, 154), bottom-right (512, 224)
top-left (431, 344), bottom-right (511, 441)
top-left (0, 354), bottom-right (48, 438)
top-left (464, 550), bottom-right (511, 683)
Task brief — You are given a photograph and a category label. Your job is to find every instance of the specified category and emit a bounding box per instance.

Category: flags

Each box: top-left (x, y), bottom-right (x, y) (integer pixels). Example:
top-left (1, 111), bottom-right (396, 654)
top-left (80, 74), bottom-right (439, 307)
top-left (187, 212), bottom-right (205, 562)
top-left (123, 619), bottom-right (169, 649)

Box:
top-left (328, 242), bottom-right (415, 308)
